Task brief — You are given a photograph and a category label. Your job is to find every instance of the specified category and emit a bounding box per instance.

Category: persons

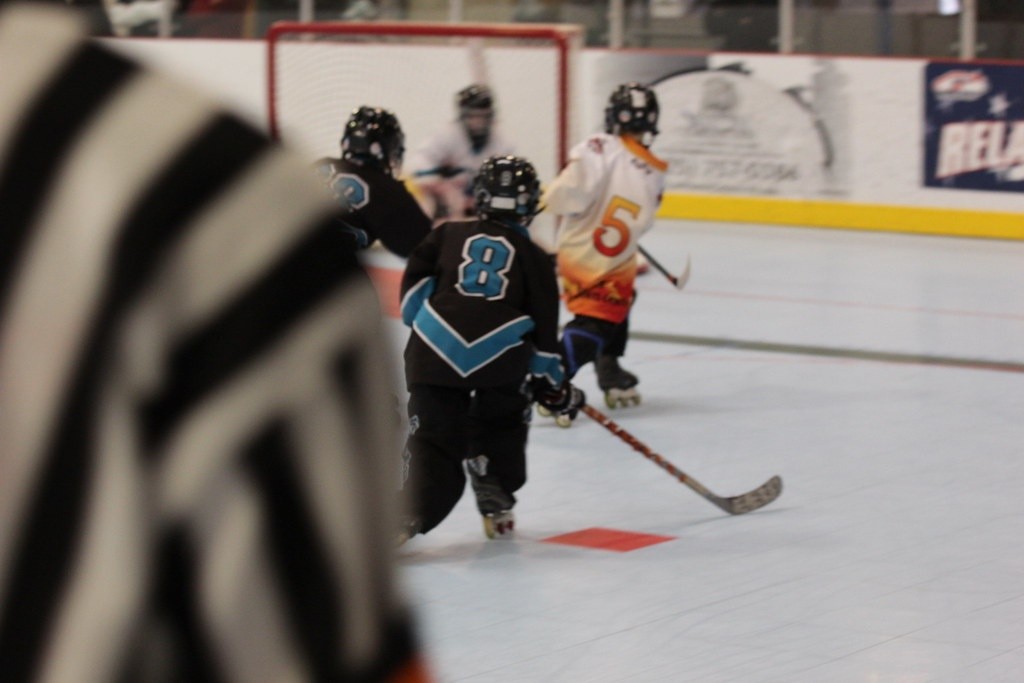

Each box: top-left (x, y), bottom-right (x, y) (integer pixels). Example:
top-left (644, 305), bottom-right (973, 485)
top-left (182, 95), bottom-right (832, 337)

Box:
top-left (1, 1), bottom-right (435, 682)
top-left (392, 156), bottom-right (559, 536)
top-left (705, 1), bottom-right (779, 52)
top-left (537, 82), bottom-right (669, 430)
top-left (311, 106), bottom-right (433, 262)
top-left (509, 0), bottom-right (651, 47)
top-left (417, 87), bottom-right (518, 219)
top-left (104, 0), bottom-right (411, 44)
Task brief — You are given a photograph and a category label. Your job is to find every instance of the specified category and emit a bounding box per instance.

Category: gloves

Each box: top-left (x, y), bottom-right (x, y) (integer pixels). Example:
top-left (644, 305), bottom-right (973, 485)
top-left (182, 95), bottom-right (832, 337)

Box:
top-left (538, 381), bottom-right (584, 418)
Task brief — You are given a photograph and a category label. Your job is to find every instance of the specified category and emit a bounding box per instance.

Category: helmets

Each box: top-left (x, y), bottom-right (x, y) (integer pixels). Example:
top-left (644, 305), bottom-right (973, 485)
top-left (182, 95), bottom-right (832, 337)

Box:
top-left (607, 80), bottom-right (660, 136)
top-left (474, 152), bottom-right (539, 228)
top-left (458, 84), bottom-right (490, 112)
top-left (340, 107), bottom-right (405, 179)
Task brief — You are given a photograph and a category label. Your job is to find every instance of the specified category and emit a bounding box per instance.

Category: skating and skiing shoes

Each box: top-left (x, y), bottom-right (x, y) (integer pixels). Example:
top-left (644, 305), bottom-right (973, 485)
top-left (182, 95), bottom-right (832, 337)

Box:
top-left (393, 514), bottom-right (423, 547)
top-left (473, 480), bottom-right (516, 538)
top-left (536, 405), bottom-right (572, 426)
top-left (598, 367), bottom-right (640, 409)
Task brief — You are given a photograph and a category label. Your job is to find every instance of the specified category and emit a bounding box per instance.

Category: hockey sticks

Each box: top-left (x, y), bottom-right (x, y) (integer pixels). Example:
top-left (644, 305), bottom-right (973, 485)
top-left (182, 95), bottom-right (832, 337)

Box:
top-left (635, 242), bottom-right (693, 290)
top-left (580, 403), bottom-right (784, 516)
